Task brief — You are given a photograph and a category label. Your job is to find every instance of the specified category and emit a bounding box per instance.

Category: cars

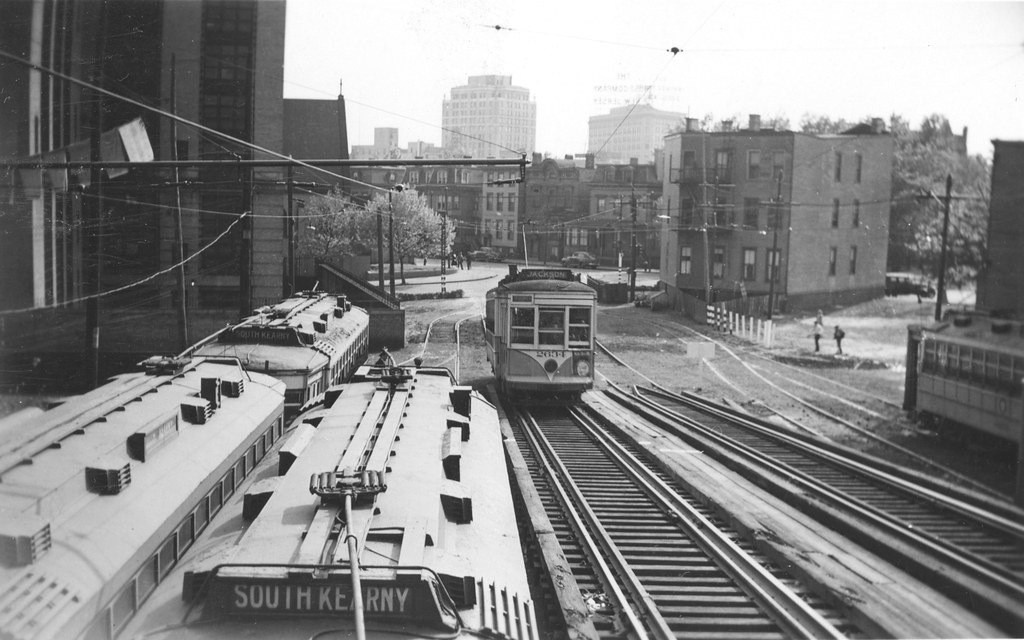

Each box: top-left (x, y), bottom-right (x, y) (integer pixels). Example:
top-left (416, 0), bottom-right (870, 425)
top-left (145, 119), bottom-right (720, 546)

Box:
top-left (885, 273), bottom-right (935, 298)
top-left (561, 252), bottom-right (599, 269)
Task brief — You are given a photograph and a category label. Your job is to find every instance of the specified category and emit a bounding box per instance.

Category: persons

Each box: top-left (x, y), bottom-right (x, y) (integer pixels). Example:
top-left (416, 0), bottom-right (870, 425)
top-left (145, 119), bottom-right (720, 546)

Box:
top-left (452, 253), bottom-right (458, 266)
top-left (814, 320), bottom-right (825, 352)
top-left (375, 351), bottom-right (388, 366)
top-left (457, 250), bottom-right (464, 269)
top-left (466, 250), bottom-right (473, 269)
top-left (424, 254), bottom-right (428, 265)
top-left (814, 307), bottom-right (824, 328)
top-left (834, 325), bottom-right (845, 354)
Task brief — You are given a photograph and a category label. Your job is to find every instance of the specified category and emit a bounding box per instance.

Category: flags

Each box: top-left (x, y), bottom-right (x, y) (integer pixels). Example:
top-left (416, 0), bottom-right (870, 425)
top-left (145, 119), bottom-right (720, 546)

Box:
top-left (100, 127), bottom-right (128, 179)
top-left (66, 136), bottom-right (93, 190)
top-left (14, 154), bottom-right (41, 200)
top-left (118, 117), bottom-right (156, 164)
top-left (0, 162), bottom-right (14, 204)
top-left (40, 146), bottom-right (69, 189)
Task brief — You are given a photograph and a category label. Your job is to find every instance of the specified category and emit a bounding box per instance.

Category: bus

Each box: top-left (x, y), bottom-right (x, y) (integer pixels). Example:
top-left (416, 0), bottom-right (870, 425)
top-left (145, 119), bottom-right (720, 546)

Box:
top-left (485, 267), bottom-right (598, 408)
top-left (0, 288), bottom-right (539, 640)
top-left (914, 309), bottom-right (1024, 458)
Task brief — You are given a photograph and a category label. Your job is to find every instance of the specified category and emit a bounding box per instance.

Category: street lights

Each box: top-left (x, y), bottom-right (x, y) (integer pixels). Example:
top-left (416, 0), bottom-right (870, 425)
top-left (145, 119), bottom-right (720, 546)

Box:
top-left (388, 184), bottom-right (404, 300)
